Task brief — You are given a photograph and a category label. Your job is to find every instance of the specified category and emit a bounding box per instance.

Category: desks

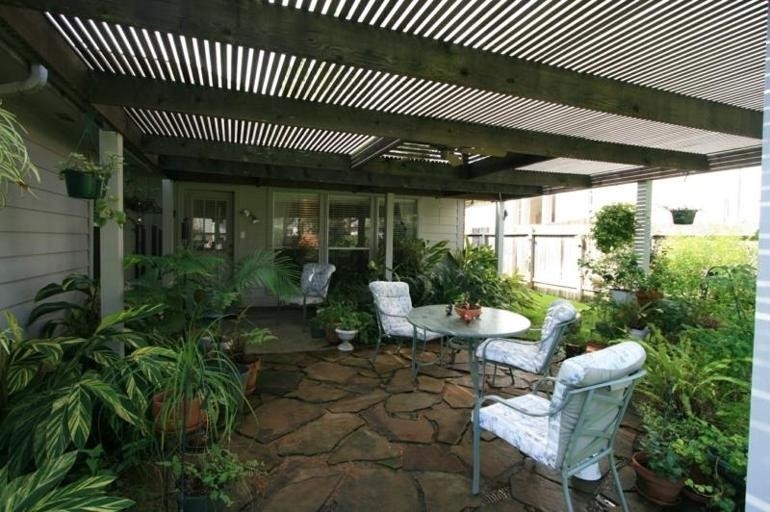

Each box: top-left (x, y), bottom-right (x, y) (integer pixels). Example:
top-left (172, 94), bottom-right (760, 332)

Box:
top-left (406, 304), bottom-right (531, 396)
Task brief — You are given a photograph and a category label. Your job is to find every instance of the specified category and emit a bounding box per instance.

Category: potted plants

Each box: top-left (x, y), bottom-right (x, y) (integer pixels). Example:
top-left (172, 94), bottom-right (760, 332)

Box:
top-left (446, 291), bottom-right (482, 320)
top-left (311, 301), bottom-right (372, 351)
top-left (0, 152), bottom-right (302, 510)
top-left (562, 203), bottom-right (751, 512)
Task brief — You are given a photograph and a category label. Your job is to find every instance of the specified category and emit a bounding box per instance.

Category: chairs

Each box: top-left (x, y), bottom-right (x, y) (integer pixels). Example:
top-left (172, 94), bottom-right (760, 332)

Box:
top-left (370, 282), bottom-right (444, 368)
top-left (470, 341), bottom-right (647, 511)
top-left (475, 300), bottom-right (581, 397)
top-left (277, 263), bottom-right (336, 331)
